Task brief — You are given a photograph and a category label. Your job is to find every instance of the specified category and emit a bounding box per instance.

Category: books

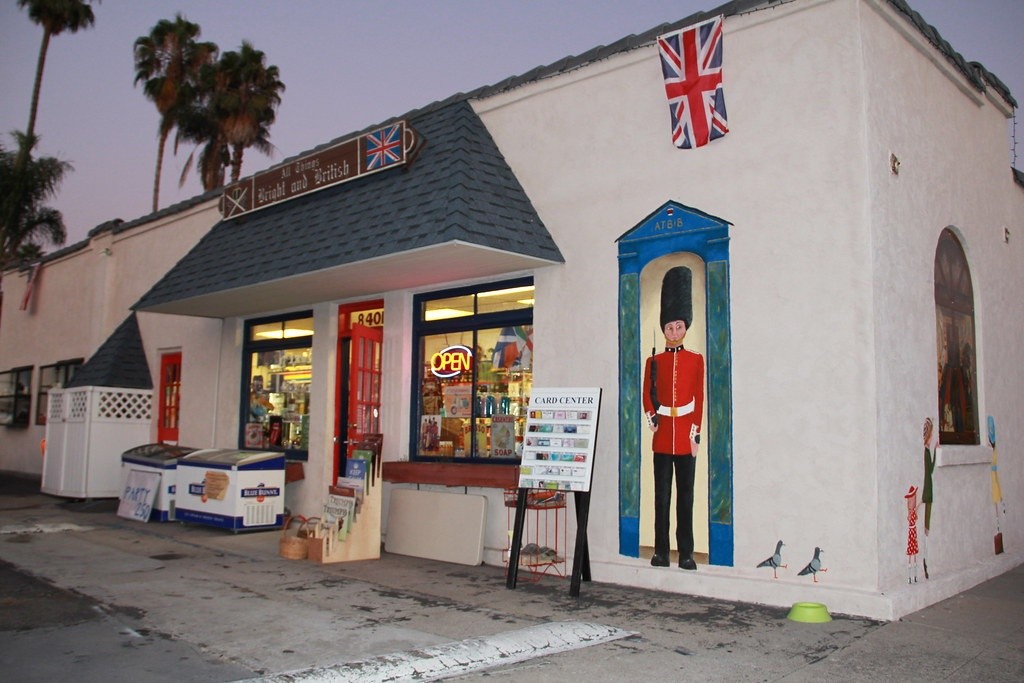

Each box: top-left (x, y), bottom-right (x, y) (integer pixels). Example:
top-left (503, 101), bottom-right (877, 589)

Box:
top-left (322, 433), bottom-right (383, 539)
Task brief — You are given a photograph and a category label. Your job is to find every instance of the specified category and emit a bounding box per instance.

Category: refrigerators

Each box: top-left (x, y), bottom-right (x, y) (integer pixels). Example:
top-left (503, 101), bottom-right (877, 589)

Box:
top-left (121, 444), bottom-right (201, 523)
top-left (175, 448), bottom-right (285, 534)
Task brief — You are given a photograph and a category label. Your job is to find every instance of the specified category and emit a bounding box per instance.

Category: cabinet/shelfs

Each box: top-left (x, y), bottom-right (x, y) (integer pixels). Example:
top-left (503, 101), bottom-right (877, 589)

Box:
top-left (501, 490), bottom-right (566, 586)
top-left (308, 432), bottom-right (384, 564)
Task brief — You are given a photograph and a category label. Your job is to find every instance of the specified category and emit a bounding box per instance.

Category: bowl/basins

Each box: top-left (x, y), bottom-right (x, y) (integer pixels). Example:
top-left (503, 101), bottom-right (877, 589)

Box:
top-left (786, 601), bottom-right (832, 623)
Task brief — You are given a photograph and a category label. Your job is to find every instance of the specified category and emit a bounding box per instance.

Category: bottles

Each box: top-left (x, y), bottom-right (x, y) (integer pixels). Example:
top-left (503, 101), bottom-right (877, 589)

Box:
top-left (439, 440), bottom-right (465, 458)
top-left (474, 383), bottom-right (520, 416)
top-left (282, 382), bottom-right (309, 419)
top-left (478, 419), bottom-right (488, 457)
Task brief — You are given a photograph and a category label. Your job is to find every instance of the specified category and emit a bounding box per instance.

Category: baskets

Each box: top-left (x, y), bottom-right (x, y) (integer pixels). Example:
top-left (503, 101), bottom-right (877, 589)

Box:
top-left (279, 513), bottom-right (311, 560)
top-left (298, 517), bottom-right (323, 561)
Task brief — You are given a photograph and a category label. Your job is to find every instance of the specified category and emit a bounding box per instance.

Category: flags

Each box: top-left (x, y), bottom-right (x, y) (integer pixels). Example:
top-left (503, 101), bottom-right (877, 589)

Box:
top-left (656, 17), bottom-right (729, 149)
top-left (493, 324), bottom-right (533, 371)
top-left (19, 263), bottom-right (42, 311)
